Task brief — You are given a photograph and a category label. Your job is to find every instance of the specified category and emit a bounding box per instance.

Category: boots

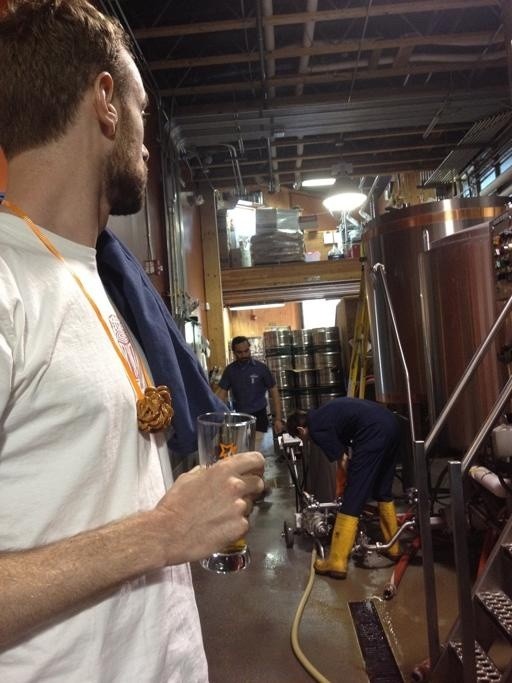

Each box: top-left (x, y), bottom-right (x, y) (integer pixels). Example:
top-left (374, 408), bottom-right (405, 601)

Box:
top-left (314, 512), bottom-right (360, 578)
top-left (378, 500), bottom-right (400, 561)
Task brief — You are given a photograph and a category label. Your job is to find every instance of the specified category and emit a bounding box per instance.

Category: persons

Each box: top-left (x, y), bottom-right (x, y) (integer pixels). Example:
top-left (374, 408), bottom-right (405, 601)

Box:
top-left (0, 0), bottom-right (265, 683)
top-left (287, 397), bottom-right (403, 579)
top-left (214, 336), bottom-right (283, 452)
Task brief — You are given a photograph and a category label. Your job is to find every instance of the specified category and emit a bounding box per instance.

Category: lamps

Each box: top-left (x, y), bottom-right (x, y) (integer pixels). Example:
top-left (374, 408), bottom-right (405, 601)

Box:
top-left (322, 162), bottom-right (367, 214)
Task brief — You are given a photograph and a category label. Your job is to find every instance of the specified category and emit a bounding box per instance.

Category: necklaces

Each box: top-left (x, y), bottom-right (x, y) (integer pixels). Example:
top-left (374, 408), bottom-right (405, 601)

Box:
top-left (0, 200), bottom-right (174, 433)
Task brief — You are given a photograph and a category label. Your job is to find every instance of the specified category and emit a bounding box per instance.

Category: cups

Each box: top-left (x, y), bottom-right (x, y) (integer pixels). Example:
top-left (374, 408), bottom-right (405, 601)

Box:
top-left (196, 411), bottom-right (257, 574)
